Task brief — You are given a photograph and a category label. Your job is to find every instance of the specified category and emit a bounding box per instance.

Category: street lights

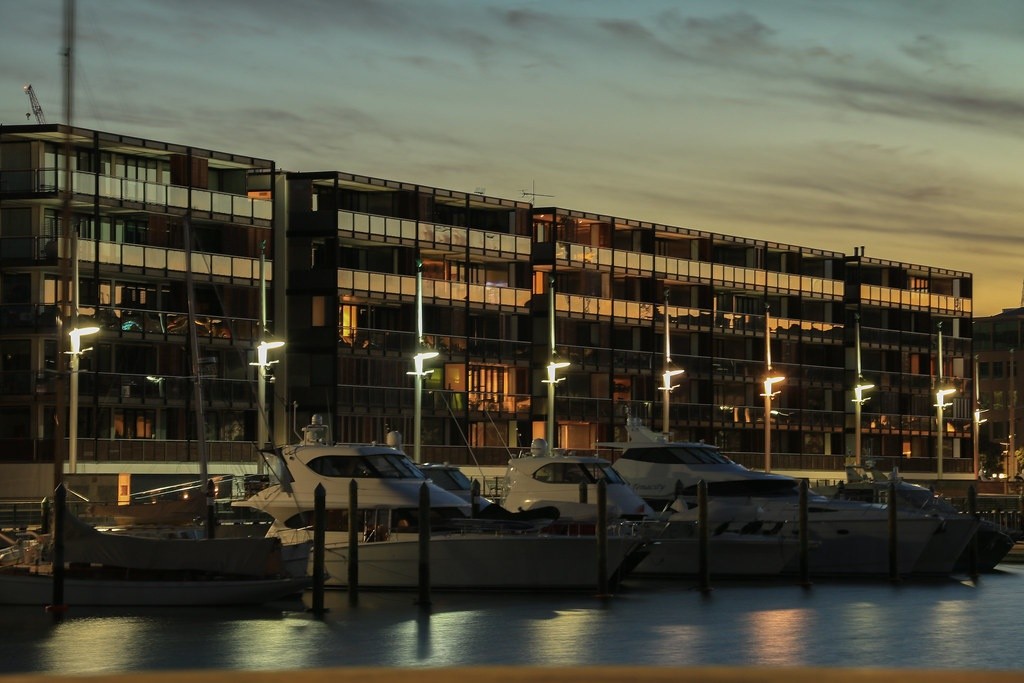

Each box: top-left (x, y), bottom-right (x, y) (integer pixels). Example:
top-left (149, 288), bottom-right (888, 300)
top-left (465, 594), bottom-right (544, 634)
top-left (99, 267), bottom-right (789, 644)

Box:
top-left (935, 386), bottom-right (958, 478)
top-left (414, 352), bottom-right (441, 463)
top-left (661, 368), bottom-right (686, 432)
top-left (854, 383), bottom-right (877, 464)
top-left (545, 361), bottom-right (572, 456)
top-left (764, 376), bottom-right (787, 471)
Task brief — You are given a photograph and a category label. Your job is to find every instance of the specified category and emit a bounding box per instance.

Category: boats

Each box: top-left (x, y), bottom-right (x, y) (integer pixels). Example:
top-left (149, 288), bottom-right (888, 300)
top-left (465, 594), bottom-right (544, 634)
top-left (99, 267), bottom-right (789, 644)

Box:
top-left (132, 399), bottom-right (1017, 592)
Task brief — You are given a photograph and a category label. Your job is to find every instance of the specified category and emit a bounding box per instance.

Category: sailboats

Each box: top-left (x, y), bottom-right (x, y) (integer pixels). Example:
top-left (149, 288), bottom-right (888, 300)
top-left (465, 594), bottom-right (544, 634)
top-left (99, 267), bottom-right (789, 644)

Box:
top-left (1, 214), bottom-right (332, 607)
